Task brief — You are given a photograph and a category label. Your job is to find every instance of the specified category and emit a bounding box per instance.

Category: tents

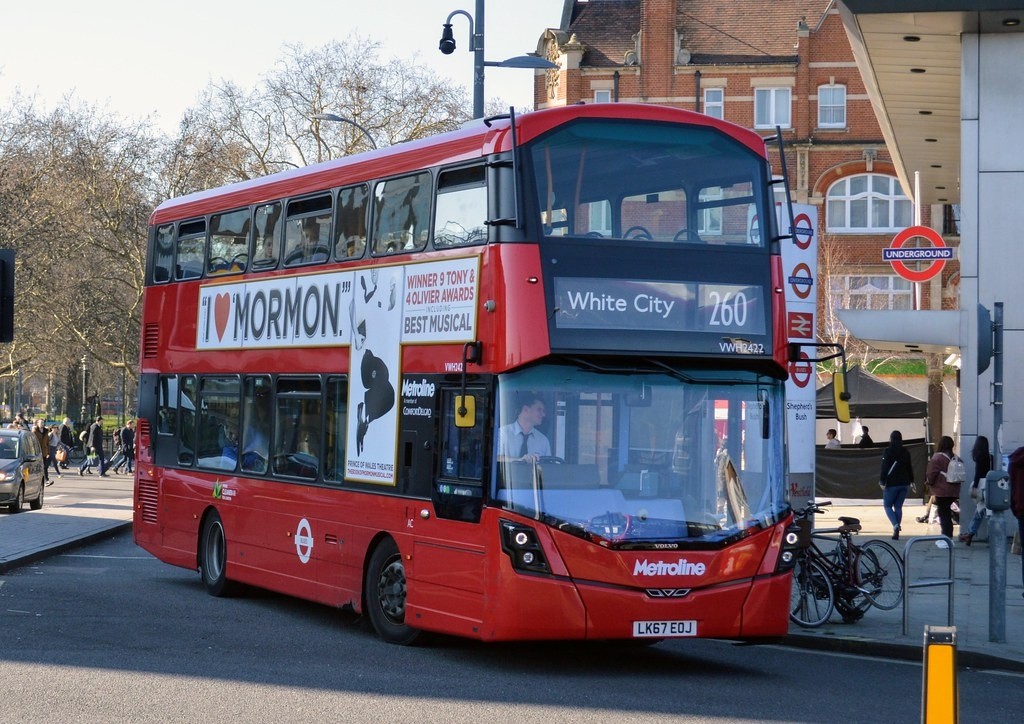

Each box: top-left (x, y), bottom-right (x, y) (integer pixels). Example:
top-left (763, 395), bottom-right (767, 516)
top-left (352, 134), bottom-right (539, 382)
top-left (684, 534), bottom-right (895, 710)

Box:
top-left (815, 365), bottom-right (929, 508)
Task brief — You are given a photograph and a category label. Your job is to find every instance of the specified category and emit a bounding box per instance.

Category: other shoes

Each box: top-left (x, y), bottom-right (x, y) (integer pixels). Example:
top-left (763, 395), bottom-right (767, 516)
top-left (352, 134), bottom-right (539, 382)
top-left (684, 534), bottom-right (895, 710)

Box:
top-left (102, 474), bottom-right (110, 477)
top-left (87, 468), bottom-right (93, 474)
top-left (915, 517), bottom-right (928, 524)
top-left (127, 472), bottom-right (135, 476)
top-left (112, 467), bottom-right (119, 475)
top-left (45, 480), bottom-right (54, 487)
top-left (77, 468), bottom-right (83, 476)
top-left (935, 538), bottom-right (955, 548)
top-left (57, 474), bottom-right (64, 479)
top-left (118, 469), bottom-right (123, 474)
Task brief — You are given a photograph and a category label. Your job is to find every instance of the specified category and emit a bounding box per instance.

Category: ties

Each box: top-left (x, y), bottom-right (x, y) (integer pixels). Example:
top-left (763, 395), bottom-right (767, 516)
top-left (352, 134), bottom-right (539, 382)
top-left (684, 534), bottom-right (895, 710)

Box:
top-left (520, 432), bottom-right (533, 457)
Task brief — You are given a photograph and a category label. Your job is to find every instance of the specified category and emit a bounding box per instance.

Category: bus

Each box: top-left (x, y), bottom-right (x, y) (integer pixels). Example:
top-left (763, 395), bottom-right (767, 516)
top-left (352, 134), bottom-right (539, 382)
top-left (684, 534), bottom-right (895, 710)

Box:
top-left (131, 100), bottom-right (856, 647)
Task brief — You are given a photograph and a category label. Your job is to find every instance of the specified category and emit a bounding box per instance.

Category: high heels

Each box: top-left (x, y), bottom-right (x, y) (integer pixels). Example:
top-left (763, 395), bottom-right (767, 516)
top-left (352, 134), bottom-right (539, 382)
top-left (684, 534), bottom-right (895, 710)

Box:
top-left (892, 524), bottom-right (901, 540)
top-left (958, 533), bottom-right (973, 546)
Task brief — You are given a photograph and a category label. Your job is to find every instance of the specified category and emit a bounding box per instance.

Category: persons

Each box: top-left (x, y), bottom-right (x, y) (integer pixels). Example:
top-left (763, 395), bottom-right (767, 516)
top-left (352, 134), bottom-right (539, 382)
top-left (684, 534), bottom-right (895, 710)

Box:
top-left (0, 404), bottom-right (136, 487)
top-left (958, 436), bottom-right (994, 547)
top-left (879, 431), bottom-right (916, 539)
top-left (916, 495), bottom-right (937, 524)
top-left (1008, 447), bottom-right (1024, 598)
top-left (497, 394), bottom-right (551, 464)
top-left (825, 429), bottom-right (841, 451)
top-left (858, 426), bottom-right (873, 445)
top-left (349, 269), bottom-right (396, 456)
top-left (251, 220), bottom-right (431, 269)
top-left (926, 435), bottom-right (964, 549)
top-left (169, 388), bottom-right (198, 436)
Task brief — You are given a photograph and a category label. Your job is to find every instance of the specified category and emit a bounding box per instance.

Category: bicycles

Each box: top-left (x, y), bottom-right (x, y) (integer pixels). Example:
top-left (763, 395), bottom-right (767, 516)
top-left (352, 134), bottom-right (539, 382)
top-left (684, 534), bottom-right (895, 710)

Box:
top-left (783, 498), bottom-right (907, 627)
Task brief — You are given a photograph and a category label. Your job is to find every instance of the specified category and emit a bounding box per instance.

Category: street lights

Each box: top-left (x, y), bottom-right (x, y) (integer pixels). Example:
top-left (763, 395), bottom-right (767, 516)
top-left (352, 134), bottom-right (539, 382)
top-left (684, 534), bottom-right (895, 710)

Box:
top-left (439, 0), bottom-right (560, 120)
top-left (79, 354), bottom-right (91, 425)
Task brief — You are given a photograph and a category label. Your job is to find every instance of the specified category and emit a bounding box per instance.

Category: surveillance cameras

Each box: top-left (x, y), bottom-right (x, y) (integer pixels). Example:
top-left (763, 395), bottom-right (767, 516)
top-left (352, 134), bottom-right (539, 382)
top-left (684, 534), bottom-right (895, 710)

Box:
top-left (438, 22), bottom-right (458, 56)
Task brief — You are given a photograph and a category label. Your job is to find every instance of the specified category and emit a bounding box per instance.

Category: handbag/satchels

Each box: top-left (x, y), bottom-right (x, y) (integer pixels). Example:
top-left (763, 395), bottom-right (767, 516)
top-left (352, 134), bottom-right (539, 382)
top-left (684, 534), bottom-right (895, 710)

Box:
top-left (122, 444), bottom-right (131, 453)
top-left (1011, 532), bottom-right (1022, 555)
top-left (969, 478), bottom-right (986, 498)
top-left (87, 451), bottom-right (99, 467)
top-left (55, 446), bottom-right (67, 462)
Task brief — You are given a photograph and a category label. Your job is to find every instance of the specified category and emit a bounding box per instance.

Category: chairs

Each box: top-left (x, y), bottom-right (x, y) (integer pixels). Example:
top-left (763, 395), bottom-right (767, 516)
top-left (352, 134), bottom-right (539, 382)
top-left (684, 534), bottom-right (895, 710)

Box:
top-left (149, 227), bottom-right (715, 279)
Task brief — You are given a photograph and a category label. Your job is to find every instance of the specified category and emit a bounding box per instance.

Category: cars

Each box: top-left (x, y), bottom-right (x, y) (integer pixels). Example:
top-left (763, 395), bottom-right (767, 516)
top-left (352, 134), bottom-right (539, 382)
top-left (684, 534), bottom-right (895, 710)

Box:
top-left (0, 428), bottom-right (46, 514)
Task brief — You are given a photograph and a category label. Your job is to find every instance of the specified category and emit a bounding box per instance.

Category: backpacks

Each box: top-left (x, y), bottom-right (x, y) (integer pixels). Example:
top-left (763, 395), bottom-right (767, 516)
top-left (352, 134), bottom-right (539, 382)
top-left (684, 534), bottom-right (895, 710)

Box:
top-left (113, 430), bottom-right (120, 445)
top-left (936, 452), bottom-right (966, 483)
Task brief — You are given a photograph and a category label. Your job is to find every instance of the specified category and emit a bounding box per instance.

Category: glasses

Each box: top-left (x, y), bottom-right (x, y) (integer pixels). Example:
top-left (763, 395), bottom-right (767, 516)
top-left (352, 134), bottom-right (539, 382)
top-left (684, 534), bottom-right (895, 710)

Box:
top-left (52, 427), bottom-right (58, 429)
top-left (264, 241), bottom-right (273, 246)
